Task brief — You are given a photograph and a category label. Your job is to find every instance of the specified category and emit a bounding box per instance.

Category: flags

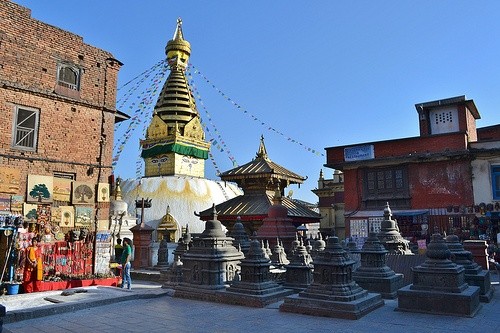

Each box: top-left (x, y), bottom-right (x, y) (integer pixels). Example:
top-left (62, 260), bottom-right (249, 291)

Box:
top-left (109, 58), bottom-right (325, 185)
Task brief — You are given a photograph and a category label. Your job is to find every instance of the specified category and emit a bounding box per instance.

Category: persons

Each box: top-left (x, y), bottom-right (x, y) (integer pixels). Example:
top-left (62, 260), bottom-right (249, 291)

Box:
top-left (12, 216), bottom-right (65, 268)
top-left (23, 237), bottom-right (40, 281)
top-left (114, 236), bottom-right (123, 249)
top-left (120, 238), bottom-right (133, 290)
